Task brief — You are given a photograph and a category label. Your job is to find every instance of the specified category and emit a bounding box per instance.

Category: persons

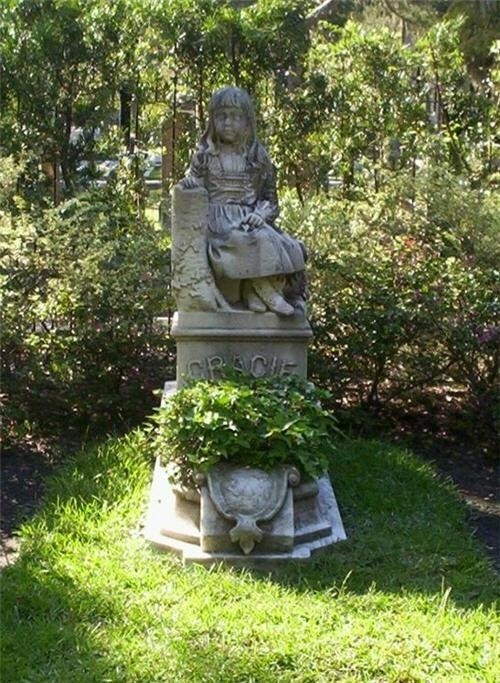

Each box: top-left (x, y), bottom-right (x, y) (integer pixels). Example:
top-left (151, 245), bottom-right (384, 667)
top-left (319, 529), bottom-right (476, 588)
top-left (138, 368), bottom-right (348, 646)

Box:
top-left (177, 86), bottom-right (305, 316)
